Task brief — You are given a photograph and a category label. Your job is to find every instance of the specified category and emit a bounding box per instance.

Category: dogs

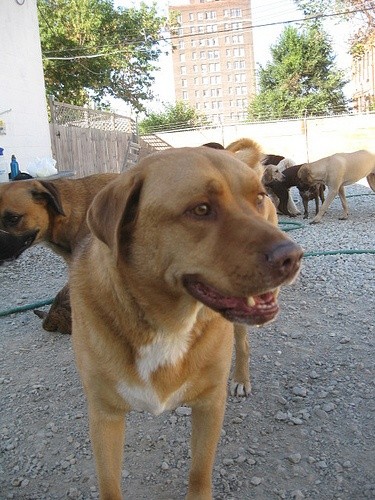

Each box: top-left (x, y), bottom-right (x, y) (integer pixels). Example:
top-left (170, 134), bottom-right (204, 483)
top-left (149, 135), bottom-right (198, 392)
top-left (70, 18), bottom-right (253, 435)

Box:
top-left (261, 148), bottom-right (375, 226)
top-left (0, 171), bottom-right (131, 335)
top-left (65, 138), bottom-right (305, 500)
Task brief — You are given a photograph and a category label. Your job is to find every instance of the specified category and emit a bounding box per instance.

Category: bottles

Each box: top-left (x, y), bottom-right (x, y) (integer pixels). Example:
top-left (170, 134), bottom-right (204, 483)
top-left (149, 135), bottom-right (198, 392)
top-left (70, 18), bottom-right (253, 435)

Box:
top-left (10, 155), bottom-right (19, 180)
top-left (0, 148), bottom-right (9, 182)
